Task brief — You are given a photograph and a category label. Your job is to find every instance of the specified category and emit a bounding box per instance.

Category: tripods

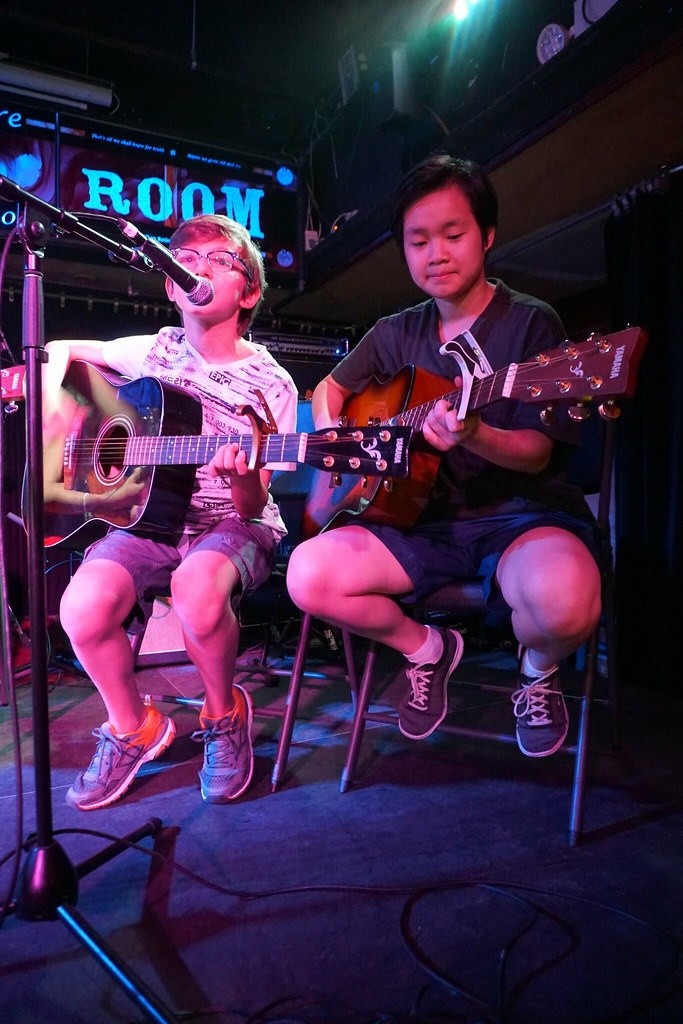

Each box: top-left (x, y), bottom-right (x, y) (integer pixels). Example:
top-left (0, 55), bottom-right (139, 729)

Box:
top-left (0, 172), bottom-right (183, 1024)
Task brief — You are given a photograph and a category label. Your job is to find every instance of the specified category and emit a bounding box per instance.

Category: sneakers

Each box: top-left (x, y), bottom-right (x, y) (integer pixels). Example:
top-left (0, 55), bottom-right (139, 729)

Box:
top-left (66, 693), bottom-right (177, 810)
top-left (511, 643), bottom-right (569, 757)
top-left (190, 683), bottom-right (254, 804)
top-left (399, 627), bottom-right (464, 740)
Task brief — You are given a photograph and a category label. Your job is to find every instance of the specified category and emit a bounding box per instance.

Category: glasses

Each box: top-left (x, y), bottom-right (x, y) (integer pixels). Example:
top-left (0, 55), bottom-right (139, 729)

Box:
top-left (171, 247), bottom-right (254, 281)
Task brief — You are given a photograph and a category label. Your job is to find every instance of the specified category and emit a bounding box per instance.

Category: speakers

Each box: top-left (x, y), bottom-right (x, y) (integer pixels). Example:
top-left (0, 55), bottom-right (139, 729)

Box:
top-left (247, 332), bottom-right (350, 404)
top-left (122, 596), bottom-right (195, 669)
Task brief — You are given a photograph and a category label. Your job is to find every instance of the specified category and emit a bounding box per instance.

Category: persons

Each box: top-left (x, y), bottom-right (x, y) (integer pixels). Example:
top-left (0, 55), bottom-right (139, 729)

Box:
top-left (27, 212), bottom-right (300, 810)
top-left (281, 153), bottom-right (603, 764)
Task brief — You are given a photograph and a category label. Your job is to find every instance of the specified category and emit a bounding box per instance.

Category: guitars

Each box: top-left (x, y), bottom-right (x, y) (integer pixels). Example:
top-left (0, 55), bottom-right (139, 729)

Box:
top-left (298, 321), bottom-right (651, 549)
top-left (20, 346), bottom-right (415, 551)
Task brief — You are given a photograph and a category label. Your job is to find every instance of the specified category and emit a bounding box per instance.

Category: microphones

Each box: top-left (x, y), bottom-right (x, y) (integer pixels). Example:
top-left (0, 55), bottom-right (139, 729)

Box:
top-left (114, 218), bottom-right (214, 308)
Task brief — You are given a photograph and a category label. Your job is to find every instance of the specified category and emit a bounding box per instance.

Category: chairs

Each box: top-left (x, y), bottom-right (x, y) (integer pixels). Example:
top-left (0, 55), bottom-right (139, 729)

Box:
top-left (124, 405), bottom-right (635, 847)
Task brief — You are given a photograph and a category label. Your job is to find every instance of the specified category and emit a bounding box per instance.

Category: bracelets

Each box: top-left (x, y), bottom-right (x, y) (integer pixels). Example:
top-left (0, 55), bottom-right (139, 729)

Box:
top-left (82, 492), bottom-right (90, 512)
